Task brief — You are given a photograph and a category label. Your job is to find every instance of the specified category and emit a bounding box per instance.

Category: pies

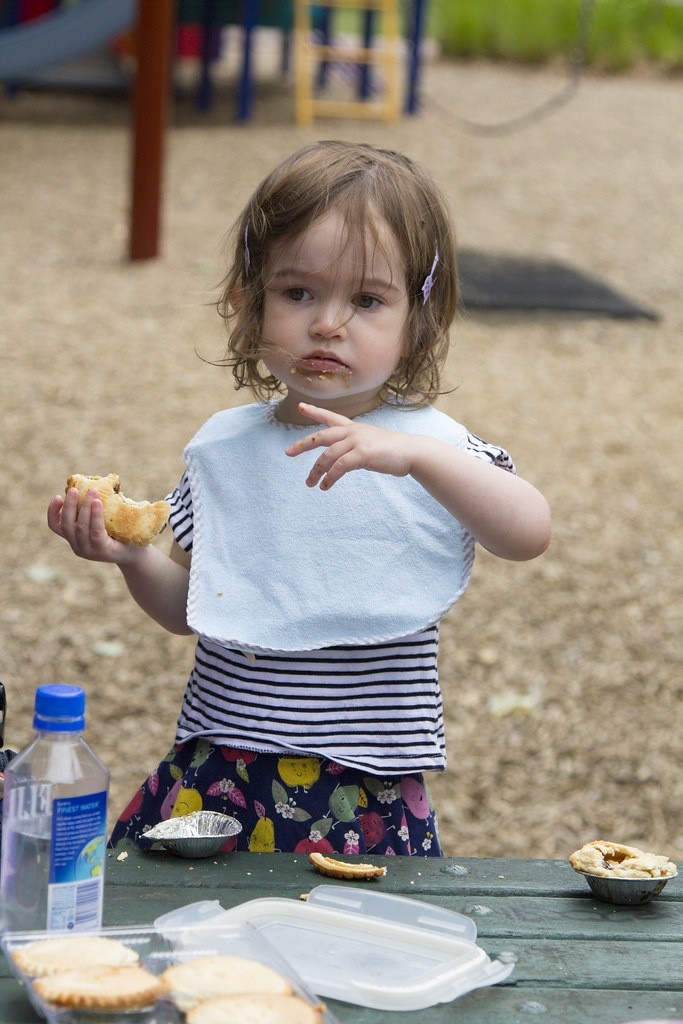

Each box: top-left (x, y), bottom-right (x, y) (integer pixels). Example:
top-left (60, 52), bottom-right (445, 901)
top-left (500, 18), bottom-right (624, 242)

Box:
top-left (65, 473), bottom-right (171, 546)
top-left (9, 936), bottom-right (323, 1024)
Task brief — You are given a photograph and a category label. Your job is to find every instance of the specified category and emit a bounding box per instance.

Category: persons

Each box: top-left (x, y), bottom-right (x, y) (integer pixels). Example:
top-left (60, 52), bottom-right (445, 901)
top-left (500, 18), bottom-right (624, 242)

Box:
top-left (45, 135), bottom-right (551, 858)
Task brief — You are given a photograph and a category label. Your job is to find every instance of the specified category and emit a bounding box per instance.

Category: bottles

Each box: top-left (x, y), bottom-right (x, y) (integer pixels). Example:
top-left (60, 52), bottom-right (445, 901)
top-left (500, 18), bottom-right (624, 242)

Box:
top-left (3, 682), bottom-right (111, 935)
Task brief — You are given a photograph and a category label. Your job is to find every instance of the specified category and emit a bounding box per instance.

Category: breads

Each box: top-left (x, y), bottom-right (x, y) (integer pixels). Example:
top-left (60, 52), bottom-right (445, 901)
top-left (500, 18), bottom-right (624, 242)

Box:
top-left (309, 852), bottom-right (386, 879)
top-left (568, 840), bottom-right (676, 878)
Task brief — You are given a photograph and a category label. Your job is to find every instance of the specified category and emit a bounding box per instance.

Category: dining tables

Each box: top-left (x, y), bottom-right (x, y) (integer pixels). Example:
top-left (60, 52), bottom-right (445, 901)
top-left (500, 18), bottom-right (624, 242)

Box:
top-left (0, 848), bottom-right (683, 1024)
